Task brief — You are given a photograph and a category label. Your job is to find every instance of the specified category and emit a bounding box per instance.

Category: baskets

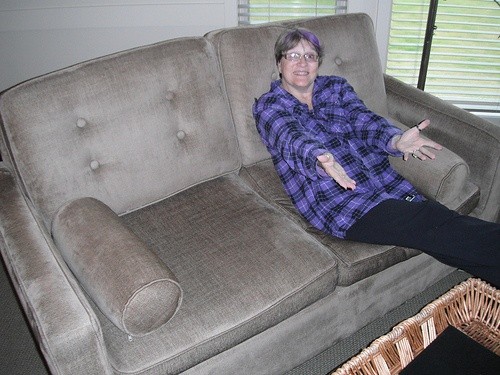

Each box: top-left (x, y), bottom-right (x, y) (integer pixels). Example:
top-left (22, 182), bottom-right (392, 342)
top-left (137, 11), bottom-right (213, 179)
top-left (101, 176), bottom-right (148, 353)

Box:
top-left (331, 278), bottom-right (500, 375)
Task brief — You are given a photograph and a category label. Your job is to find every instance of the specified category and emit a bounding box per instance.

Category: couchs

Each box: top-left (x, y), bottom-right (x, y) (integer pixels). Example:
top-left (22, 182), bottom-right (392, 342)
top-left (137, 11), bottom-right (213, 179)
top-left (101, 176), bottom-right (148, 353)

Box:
top-left (0, 14), bottom-right (500, 375)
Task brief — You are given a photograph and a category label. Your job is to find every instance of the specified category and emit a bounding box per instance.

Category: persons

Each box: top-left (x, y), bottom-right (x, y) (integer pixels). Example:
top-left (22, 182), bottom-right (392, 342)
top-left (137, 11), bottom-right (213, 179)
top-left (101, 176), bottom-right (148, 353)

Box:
top-left (252, 27), bottom-right (500, 290)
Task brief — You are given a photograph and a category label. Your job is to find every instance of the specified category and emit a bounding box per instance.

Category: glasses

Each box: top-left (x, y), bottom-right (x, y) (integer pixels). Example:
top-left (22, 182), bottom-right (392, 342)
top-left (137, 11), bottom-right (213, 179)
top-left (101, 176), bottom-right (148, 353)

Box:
top-left (281, 53), bottom-right (319, 62)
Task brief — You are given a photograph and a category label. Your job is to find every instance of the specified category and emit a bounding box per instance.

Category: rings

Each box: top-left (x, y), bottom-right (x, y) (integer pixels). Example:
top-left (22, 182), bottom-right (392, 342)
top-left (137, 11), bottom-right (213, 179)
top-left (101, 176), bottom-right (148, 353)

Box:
top-left (416, 124), bottom-right (422, 131)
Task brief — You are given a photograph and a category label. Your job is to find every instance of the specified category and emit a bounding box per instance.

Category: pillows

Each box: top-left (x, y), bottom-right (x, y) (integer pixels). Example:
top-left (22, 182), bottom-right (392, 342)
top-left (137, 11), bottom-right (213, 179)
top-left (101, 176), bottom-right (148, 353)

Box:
top-left (53, 197), bottom-right (182, 337)
top-left (378, 116), bottom-right (471, 201)
